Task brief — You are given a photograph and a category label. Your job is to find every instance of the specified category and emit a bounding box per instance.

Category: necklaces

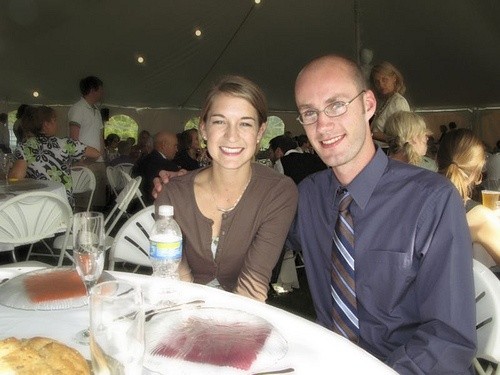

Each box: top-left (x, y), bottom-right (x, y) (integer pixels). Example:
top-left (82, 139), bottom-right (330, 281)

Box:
top-left (209, 164), bottom-right (253, 212)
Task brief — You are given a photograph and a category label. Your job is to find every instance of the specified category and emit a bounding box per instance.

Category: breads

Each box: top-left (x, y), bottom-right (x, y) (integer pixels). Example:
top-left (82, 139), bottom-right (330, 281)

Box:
top-left (0, 336), bottom-right (91, 375)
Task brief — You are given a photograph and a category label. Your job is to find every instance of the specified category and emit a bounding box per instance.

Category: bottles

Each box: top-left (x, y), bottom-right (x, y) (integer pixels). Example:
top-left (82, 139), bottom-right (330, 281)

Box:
top-left (149, 205), bottom-right (182, 305)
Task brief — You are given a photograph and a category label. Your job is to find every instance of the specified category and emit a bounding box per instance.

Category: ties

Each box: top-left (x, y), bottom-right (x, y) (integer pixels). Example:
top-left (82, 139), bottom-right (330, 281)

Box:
top-left (331, 191), bottom-right (359, 344)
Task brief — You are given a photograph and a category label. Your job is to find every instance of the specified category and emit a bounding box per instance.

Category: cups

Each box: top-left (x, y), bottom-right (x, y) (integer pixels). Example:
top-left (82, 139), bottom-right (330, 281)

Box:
top-left (481, 190), bottom-right (500, 211)
top-left (89, 281), bottom-right (145, 375)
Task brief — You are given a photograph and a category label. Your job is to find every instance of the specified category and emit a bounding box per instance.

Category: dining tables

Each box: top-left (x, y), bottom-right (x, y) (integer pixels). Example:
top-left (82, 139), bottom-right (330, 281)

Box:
top-left (0, 266), bottom-right (401, 375)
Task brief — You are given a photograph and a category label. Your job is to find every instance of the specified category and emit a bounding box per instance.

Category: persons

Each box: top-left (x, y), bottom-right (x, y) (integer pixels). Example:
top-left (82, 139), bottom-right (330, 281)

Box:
top-left (8, 104), bottom-right (100, 213)
top-left (131, 131), bottom-right (184, 215)
top-left (68, 76), bottom-right (105, 212)
top-left (267, 132), bottom-right (328, 185)
top-left (155, 75), bottom-right (298, 302)
top-left (384, 111), bottom-right (438, 171)
top-left (371, 60), bottom-right (410, 157)
top-left (152, 54), bottom-right (477, 375)
top-left (0, 104), bottom-right (28, 153)
top-left (440, 122), bottom-right (456, 142)
top-left (438, 128), bottom-right (500, 268)
top-left (174, 129), bottom-right (205, 170)
top-left (102, 131), bottom-right (151, 164)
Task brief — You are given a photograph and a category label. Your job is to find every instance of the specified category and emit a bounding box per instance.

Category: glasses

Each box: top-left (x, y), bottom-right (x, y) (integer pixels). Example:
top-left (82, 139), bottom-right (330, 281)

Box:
top-left (296, 90), bottom-right (366, 125)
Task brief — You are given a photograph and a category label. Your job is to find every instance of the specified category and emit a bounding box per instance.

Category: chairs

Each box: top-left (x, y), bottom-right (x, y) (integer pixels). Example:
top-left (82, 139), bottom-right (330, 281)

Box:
top-left (0, 161), bottom-right (158, 275)
top-left (471, 258), bottom-right (500, 375)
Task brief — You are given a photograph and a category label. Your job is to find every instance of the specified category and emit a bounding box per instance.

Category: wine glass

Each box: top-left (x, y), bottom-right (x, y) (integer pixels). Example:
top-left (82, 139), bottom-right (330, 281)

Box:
top-left (71, 212), bottom-right (105, 346)
top-left (0, 154), bottom-right (13, 187)
